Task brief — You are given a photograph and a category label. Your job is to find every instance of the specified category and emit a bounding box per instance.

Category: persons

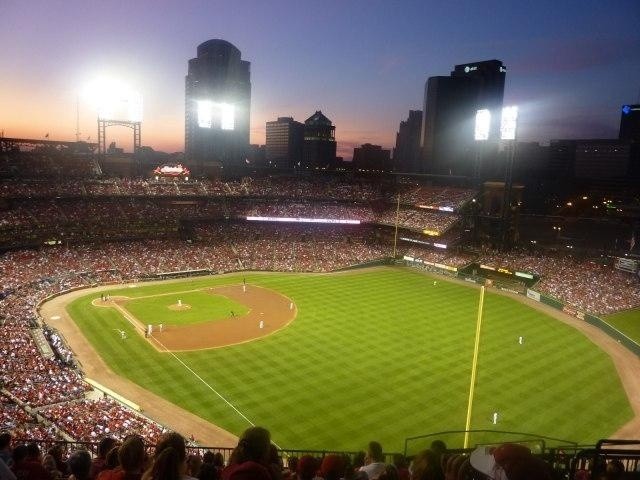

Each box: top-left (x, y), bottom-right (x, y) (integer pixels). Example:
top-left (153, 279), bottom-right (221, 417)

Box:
top-left (243, 278), bottom-right (246, 284)
top-left (243, 284), bottom-right (246, 293)
top-left (204, 425), bottom-right (640, 479)
top-left (2, 145), bottom-right (496, 275)
top-left (289, 302), bottom-right (293, 310)
top-left (230, 311), bottom-right (234, 317)
top-left (433, 279), bottom-right (437, 286)
top-left (0, 274), bottom-right (204, 480)
top-left (492, 412), bottom-right (498, 424)
top-left (494, 235), bottom-right (639, 319)
top-left (260, 319), bottom-right (264, 329)
top-left (519, 335), bottom-right (523, 345)
